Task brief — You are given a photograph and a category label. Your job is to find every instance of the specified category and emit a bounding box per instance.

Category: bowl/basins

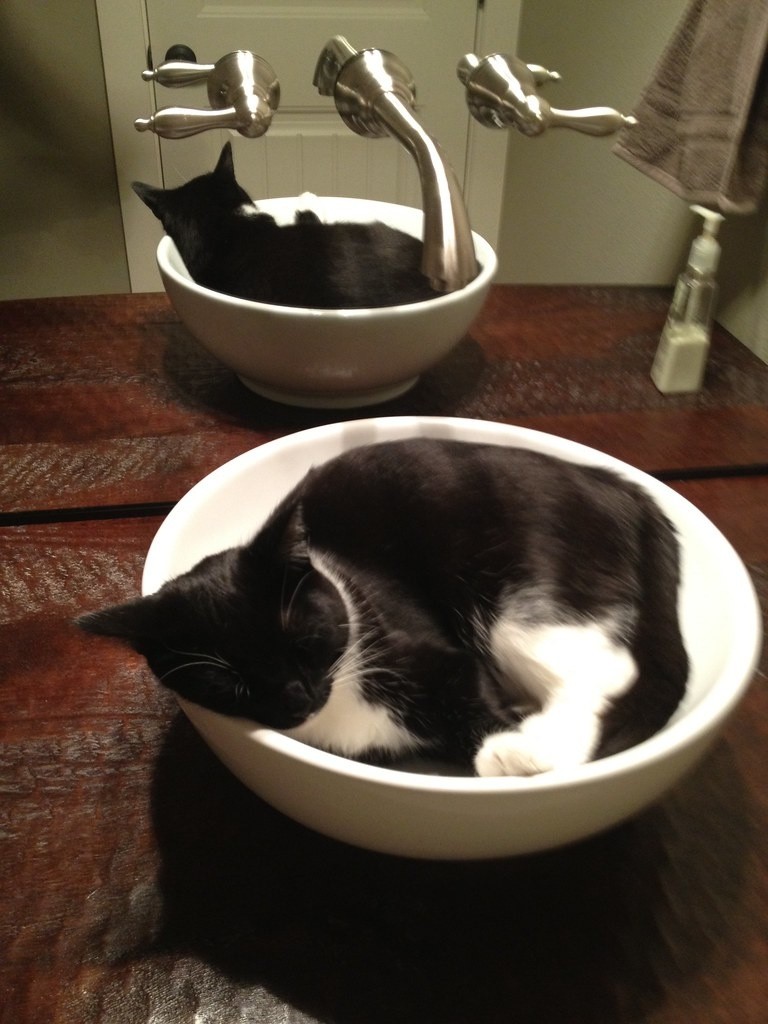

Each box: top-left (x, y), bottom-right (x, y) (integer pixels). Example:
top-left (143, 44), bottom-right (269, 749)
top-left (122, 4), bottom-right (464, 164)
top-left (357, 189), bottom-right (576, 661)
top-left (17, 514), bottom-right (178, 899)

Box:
top-left (139, 415), bottom-right (766, 862)
top-left (156, 196), bottom-right (501, 411)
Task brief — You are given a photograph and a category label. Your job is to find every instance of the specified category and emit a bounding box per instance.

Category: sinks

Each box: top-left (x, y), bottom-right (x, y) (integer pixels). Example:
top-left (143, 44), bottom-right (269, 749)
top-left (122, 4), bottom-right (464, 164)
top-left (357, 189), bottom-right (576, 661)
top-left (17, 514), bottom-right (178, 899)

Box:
top-left (156, 197), bottom-right (501, 411)
top-left (138, 412), bottom-right (763, 866)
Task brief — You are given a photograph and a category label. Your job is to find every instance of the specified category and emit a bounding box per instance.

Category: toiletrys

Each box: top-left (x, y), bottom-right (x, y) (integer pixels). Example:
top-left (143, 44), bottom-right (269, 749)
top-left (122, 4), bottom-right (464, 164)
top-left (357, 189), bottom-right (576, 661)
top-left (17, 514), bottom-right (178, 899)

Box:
top-left (650, 205), bottom-right (726, 398)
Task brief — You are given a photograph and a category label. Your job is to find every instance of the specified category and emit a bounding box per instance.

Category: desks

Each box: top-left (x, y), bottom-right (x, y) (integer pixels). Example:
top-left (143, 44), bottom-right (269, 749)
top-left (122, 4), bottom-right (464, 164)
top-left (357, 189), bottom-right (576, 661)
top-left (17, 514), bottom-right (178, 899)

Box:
top-left (0, 240), bottom-right (765, 513)
top-left (0, 469), bottom-right (768, 1024)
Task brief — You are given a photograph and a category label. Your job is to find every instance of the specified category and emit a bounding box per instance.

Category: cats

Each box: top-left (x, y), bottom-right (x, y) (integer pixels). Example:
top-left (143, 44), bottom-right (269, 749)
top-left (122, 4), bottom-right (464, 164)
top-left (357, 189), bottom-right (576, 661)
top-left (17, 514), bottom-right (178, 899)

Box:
top-left (130, 139), bottom-right (449, 309)
top-left (76, 436), bottom-right (691, 777)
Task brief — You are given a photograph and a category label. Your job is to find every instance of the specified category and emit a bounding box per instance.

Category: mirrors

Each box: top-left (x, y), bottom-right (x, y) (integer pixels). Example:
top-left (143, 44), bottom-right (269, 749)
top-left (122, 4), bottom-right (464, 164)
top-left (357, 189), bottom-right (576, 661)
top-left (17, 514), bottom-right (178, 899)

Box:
top-left (0, 1), bottom-right (768, 514)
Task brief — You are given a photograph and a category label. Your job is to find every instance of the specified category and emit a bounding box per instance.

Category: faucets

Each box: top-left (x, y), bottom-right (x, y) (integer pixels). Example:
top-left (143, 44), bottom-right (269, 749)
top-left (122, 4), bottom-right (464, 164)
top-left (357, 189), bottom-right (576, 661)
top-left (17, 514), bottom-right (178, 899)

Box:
top-left (331, 48), bottom-right (481, 293)
top-left (312, 34), bottom-right (481, 292)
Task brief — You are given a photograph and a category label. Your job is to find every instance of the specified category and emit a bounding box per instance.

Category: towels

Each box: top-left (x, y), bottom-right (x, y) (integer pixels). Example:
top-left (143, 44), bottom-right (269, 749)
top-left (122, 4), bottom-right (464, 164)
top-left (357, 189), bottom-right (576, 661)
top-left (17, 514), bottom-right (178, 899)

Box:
top-left (610, 0), bottom-right (767, 215)
